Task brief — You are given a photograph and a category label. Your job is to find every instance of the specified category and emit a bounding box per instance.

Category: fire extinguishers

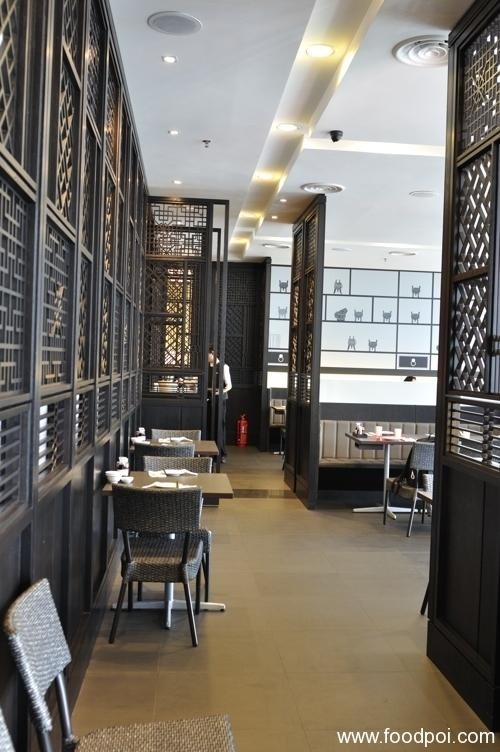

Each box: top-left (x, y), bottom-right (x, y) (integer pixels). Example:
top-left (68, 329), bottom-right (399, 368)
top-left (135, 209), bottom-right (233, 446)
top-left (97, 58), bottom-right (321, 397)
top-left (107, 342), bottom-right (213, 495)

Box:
top-left (236, 414), bottom-right (249, 448)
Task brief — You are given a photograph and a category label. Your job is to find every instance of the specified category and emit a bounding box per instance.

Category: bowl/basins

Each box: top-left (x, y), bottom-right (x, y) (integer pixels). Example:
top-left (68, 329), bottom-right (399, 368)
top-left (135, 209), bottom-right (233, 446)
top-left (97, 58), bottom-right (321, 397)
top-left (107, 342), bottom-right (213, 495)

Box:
top-left (102, 455), bottom-right (135, 485)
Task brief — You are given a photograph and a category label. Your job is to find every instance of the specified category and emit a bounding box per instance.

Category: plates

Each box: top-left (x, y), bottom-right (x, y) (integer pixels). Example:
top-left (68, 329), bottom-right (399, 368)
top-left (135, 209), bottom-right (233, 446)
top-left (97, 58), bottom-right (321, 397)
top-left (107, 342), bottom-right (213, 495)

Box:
top-left (152, 372), bottom-right (198, 394)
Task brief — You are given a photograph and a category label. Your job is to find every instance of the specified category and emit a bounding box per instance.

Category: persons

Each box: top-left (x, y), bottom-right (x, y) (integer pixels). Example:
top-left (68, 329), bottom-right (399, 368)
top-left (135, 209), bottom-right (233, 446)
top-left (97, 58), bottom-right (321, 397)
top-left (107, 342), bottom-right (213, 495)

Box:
top-left (207, 346), bottom-right (233, 464)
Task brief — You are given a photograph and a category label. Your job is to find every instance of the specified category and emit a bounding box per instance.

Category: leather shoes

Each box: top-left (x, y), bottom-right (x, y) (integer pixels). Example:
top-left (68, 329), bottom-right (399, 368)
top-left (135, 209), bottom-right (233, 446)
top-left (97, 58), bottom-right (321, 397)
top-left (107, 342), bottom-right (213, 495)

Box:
top-left (221, 457), bottom-right (226, 464)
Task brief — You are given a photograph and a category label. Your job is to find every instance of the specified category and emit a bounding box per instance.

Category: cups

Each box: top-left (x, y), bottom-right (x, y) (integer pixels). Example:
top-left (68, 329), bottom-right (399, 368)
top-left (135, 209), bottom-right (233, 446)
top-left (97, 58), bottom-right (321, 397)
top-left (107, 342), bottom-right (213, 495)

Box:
top-left (463, 431), bottom-right (471, 439)
top-left (351, 420), bottom-right (405, 440)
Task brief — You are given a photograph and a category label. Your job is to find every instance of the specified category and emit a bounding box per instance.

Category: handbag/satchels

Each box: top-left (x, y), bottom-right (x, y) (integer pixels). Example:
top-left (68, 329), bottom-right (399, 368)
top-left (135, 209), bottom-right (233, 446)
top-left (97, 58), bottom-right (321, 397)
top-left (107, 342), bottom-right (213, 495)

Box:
top-left (390, 447), bottom-right (429, 497)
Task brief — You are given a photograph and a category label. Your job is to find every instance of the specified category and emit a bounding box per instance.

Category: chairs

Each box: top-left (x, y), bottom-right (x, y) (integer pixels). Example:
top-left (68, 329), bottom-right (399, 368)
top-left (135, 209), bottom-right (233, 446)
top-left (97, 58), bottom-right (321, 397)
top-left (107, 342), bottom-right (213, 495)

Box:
top-left (2, 577), bottom-right (237, 752)
top-left (383, 442), bottom-right (437, 536)
top-left (102, 429), bottom-right (234, 647)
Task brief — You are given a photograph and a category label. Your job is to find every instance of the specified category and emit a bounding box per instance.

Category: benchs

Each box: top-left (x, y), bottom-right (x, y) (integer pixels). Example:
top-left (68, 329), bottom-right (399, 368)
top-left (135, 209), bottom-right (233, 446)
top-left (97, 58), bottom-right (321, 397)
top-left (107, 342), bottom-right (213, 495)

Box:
top-left (319, 420), bottom-right (500, 469)
top-left (270, 399), bottom-right (287, 426)
